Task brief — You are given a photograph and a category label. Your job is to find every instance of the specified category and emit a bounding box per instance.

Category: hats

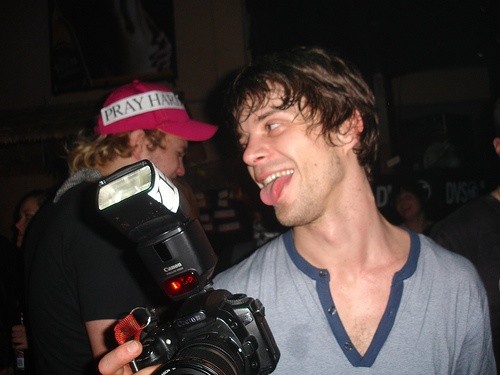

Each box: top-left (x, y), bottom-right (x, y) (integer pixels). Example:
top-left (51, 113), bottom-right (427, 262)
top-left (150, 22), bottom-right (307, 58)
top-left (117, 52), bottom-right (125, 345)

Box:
top-left (93, 79), bottom-right (218, 141)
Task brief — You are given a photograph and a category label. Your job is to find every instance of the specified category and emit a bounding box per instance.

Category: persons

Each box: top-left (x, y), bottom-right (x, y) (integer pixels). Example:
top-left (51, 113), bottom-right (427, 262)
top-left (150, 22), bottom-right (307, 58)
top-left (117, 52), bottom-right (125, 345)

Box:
top-left (0, 191), bottom-right (53, 375)
top-left (98, 46), bottom-right (497, 375)
top-left (391, 106), bottom-right (500, 371)
top-left (21, 80), bottom-right (218, 375)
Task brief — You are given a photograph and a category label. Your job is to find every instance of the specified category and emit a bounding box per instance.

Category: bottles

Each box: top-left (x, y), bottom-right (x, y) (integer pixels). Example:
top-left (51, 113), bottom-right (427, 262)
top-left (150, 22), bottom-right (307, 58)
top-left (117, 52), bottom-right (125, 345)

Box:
top-left (14, 312), bottom-right (28, 375)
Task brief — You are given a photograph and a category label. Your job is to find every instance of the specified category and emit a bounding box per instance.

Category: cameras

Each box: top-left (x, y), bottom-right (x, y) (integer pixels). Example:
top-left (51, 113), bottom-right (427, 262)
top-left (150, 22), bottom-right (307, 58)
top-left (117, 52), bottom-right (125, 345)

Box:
top-left (90, 158), bottom-right (280, 375)
